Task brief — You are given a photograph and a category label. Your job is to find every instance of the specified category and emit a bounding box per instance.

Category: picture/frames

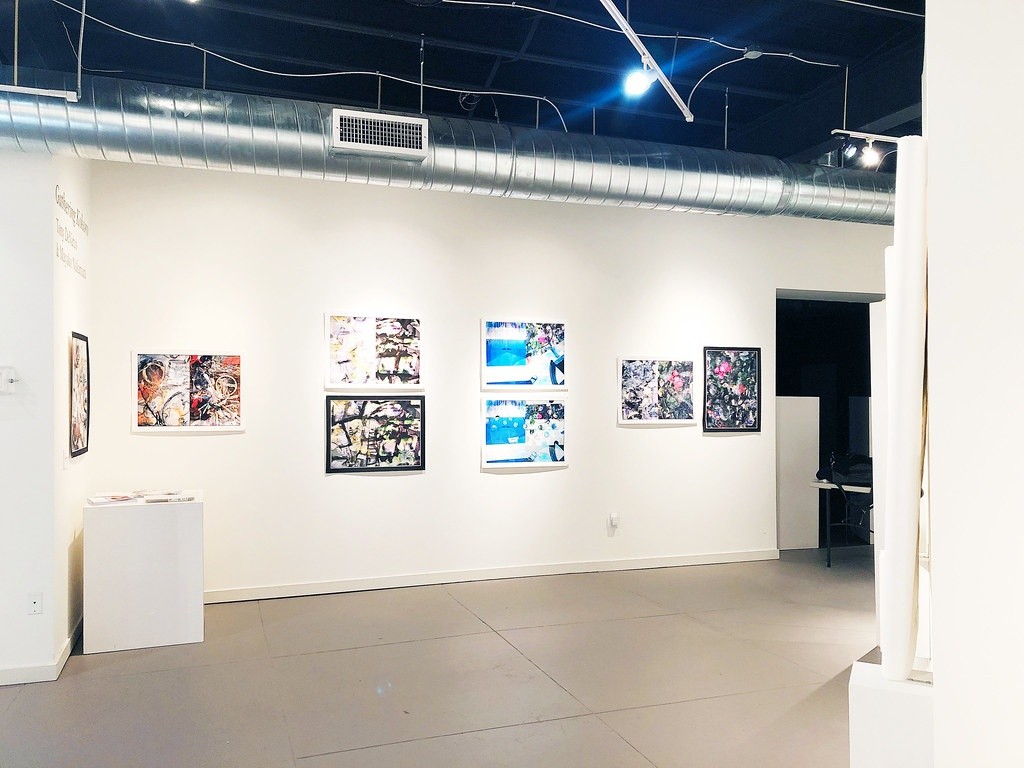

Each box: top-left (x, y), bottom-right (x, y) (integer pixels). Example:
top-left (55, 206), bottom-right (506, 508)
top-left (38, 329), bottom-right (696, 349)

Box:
top-left (618, 356), bottom-right (698, 425)
top-left (703, 347), bottom-right (761, 432)
top-left (481, 397), bottom-right (569, 468)
top-left (326, 395), bottom-right (425, 473)
top-left (132, 350), bottom-right (245, 432)
top-left (481, 319), bottom-right (568, 391)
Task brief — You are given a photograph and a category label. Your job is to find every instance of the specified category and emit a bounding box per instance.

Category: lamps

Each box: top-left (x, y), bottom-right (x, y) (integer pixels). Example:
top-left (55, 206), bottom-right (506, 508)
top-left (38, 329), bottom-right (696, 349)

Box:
top-left (838, 135), bottom-right (857, 157)
top-left (625, 53), bottom-right (658, 95)
top-left (861, 137), bottom-right (879, 163)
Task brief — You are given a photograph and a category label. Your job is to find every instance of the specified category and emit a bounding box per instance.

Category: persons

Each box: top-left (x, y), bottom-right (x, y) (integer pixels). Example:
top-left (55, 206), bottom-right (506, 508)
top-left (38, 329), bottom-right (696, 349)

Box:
top-left (535, 325), bottom-right (558, 355)
top-left (537, 405), bottom-right (544, 420)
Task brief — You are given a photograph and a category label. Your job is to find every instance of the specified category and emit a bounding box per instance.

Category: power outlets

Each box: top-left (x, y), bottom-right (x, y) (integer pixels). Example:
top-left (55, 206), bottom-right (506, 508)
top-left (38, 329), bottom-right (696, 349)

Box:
top-left (25, 592), bottom-right (41, 614)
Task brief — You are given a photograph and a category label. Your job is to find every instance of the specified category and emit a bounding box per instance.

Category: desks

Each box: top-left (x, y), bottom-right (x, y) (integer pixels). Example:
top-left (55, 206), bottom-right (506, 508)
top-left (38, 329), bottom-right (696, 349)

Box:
top-left (808, 480), bottom-right (873, 567)
top-left (82, 492), bottom-right (204, 654)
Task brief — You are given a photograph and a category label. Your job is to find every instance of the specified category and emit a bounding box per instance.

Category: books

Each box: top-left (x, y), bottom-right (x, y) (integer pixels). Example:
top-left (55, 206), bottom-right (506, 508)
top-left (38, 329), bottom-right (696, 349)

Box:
top-left (144, 495), bottom-right (195, 503)
top-left (130, 490), bottom-right (182, 498)
top-left (86, 496), bottom-right (138, 506)
